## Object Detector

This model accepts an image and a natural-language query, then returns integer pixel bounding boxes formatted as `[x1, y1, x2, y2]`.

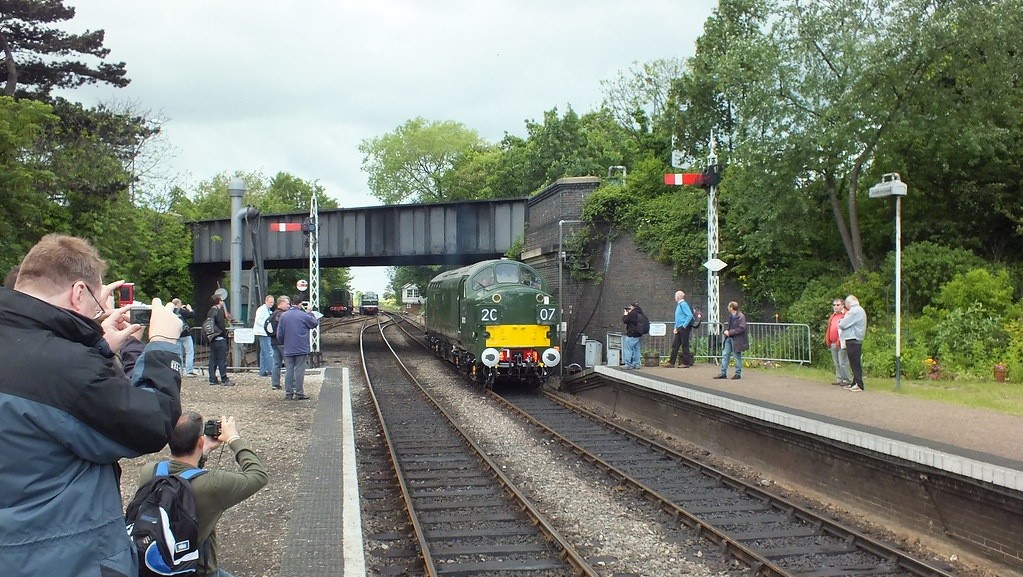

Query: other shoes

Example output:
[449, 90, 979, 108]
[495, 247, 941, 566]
[663, 363, 674, 368]
[223, 381, 238, 385]
[188, 373, 197, 376]
[832, 382, 841, 385]
[296, 395, 309, 400]
[622, 366, 631, 369]
[209, 381, 218, 385]
[844, 385, 851, 389]
[272, 385, 281, 389]
[840, 383, 848, 386]
[632, 366, 639, 369]
[263, 372, 267, 376]
[286, 396, 292, 400]
[678, 364, 689, 368]
[732, 375, 740, 379]
[713, 374, 725, 379]
[850, 384, 861, 391]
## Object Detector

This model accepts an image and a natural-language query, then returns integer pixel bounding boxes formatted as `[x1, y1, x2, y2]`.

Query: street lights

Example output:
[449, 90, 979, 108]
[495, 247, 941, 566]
[869, 173, 907, 391]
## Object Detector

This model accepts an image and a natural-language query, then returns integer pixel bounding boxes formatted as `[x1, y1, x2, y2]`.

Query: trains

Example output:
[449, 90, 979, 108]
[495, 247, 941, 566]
[422, 258, 562, 392]
[359, 291, 379, 316]
[326, 288, 354, 316]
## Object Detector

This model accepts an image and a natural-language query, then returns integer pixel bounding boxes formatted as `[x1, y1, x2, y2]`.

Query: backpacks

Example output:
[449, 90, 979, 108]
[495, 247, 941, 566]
[176, 308, 189, 332]
[126, 461, 209, 577]
[631, 310, 648, 334]
[202, 309, 224, 342]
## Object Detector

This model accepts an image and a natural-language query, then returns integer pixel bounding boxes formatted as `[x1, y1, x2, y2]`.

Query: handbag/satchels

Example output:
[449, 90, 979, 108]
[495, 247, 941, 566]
[680, 301, 703, 328]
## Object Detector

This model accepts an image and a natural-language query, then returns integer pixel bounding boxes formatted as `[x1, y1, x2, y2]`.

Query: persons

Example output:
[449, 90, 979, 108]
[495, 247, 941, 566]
[621, 302, 642, 370]
[277, 296, 318, 401]
[0, 235, 184, 577]
[713, 302, 749, 379]
[253, 295, 274, 376]
[838, 295, 866, 391]
[269, 296, 296, 390]
[207, 294, 235, 386]
[662, 291, 693, 368]
[4, 264, 146, 379]
[137, 411, 269, 577]
[825, 299, 850, 386]
[171, 298, 199, 376]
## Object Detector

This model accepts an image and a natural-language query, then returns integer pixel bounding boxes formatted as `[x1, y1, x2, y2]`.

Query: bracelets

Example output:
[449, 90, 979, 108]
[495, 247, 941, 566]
[227, 436, 240, 445]
[147, 335, 181, 341]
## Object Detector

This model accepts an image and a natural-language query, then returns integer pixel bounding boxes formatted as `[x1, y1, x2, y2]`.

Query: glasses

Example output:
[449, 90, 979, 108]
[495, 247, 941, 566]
[73, 284, 104, 320]
[833, 304, 841, 306]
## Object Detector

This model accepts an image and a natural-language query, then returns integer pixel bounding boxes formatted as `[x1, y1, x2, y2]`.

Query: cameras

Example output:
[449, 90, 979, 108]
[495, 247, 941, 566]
[130, 307, 151, 326]
[203, 420, 223, 435]
[625, 308, 631, 311]
[118, 283, 135, 307]
